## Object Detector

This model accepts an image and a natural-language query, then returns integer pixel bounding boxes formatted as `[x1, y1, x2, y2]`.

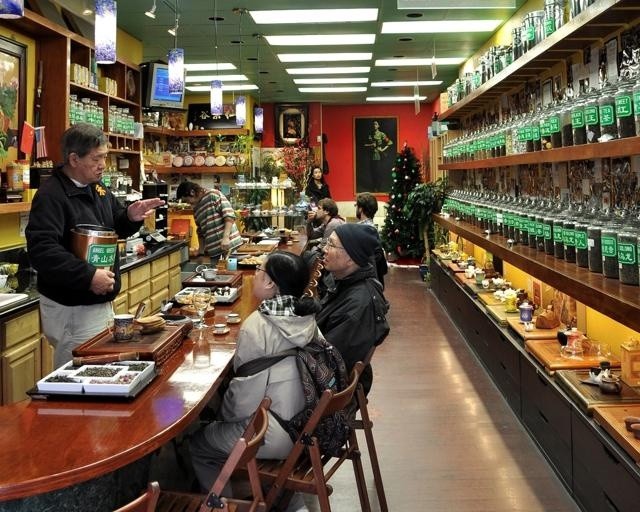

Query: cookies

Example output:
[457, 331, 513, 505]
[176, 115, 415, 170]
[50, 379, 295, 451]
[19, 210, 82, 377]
[137, 317, 166, 335]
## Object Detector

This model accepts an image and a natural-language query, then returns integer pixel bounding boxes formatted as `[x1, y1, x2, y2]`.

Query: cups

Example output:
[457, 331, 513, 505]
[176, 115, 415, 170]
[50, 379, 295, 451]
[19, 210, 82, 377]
[555, 326, 611, 361]
[105, 314, 135, 342]
[229, 314, 239, 322]
[292, 231, 299, 242]
[252, 210, 286, 217]
[215, 323, 225, 333]
[631, 423, 640, 440]
[287, 241, 293, 245]
[623, 415, 640, 431]
[589, 361, 610, 382]
[204, 270, 216, 281]
[227, 258, 237, 271]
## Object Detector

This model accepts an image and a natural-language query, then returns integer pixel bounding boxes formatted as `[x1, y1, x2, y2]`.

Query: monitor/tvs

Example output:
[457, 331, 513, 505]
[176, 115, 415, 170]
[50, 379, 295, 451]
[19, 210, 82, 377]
[137, 60, 185, 110]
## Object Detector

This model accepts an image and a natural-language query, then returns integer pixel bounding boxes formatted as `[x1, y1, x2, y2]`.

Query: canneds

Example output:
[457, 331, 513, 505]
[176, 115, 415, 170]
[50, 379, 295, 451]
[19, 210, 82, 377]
[6, 165, 24, 192]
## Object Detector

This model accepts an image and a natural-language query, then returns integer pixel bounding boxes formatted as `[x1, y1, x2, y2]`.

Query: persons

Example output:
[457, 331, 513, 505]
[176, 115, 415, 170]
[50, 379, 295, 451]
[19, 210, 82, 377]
[354, 192, 379, 228]
[175, 179, 244, 266]
[292, 223, 390, 409]
[286, 116, 300, 137]
[305, 165, 333, 219]
[305, 200, 343, 267]
[25, 124, 167, 373]
[363, 121, 394, 174]
[188, 251, 341, 498]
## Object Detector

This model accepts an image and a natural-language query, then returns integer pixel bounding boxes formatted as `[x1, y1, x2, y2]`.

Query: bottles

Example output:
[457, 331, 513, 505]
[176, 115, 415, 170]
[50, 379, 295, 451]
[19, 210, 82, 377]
[100, 166, 132, 194]
[446, 188, 640, 289]
[14, 160, 30, 189]
[218, 259, 225, 270]
[443, 0, 640, 166]
[71, 94, 135, 137]
[619, 339, 640, 388]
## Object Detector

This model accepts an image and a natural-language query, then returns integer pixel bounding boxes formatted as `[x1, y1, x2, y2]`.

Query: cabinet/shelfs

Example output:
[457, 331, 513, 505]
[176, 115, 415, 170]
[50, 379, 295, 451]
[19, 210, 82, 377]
[143, 125, 252, 174]
[425, 1, 640, 510]
[0, 244, 182, 396]
[41, 37, 141, 185]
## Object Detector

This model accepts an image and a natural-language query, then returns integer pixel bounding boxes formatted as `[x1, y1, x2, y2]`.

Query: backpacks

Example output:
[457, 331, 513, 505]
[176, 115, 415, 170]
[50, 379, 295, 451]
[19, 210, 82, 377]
[231, 312, 358, 457]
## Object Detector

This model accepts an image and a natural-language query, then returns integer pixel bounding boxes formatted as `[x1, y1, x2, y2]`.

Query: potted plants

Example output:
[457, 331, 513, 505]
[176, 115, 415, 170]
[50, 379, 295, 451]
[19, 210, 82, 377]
[380, 140, 427, 269]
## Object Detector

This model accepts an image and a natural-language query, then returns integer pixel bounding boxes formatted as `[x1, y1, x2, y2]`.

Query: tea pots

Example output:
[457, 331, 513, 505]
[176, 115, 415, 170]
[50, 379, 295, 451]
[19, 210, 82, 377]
[599, 373, 622, 395]
[493, 289, 537, 323]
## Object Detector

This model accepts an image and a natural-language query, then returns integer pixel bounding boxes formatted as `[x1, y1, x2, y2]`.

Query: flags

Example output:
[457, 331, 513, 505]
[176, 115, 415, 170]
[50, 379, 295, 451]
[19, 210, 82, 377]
[34, 127, 48, 159]
[20, 121, 35, 155]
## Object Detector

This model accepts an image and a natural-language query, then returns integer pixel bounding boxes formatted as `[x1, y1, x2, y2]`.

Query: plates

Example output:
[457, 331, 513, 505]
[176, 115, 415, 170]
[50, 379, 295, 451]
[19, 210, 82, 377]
[36, 359, 155, 395]
[237, 261, 260, 269]
[578, 373, 621, 386]
[212, 328, 230, 335]
[227, 318, 241, 324]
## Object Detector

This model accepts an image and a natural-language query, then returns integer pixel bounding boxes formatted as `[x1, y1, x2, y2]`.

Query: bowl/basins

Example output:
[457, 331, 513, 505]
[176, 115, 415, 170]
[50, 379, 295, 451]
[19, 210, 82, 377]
[0, 261, 21, 291]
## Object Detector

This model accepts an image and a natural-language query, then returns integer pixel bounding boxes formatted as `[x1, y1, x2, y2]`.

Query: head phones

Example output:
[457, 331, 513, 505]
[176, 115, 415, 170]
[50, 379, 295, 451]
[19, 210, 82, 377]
[189, 186, 195, 196]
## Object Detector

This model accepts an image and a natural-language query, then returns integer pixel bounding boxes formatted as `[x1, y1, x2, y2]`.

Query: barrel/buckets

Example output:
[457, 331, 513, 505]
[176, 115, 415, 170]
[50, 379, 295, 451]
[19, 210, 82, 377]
[69, 222, 119, 273]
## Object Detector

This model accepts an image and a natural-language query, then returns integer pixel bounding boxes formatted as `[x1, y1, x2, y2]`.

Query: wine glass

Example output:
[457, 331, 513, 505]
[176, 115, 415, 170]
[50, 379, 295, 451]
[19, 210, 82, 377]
[192, 287, 212, 328]
[192, 328, 212, 369]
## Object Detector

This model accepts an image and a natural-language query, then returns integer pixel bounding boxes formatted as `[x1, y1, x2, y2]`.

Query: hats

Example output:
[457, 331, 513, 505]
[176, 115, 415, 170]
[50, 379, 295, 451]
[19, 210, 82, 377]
[334, 222, 380, 268]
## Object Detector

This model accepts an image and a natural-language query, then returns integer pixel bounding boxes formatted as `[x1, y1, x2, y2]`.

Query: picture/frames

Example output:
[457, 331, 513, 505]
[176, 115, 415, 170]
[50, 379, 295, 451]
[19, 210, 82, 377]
[274, 102, 310, 148]
[353, 116, 401, 197]
[0, 35, 28, 181]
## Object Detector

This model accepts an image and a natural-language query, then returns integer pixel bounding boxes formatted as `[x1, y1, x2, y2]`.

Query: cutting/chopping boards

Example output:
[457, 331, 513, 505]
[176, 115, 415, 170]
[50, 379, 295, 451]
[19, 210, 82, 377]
[237, 243, 275, 252]
